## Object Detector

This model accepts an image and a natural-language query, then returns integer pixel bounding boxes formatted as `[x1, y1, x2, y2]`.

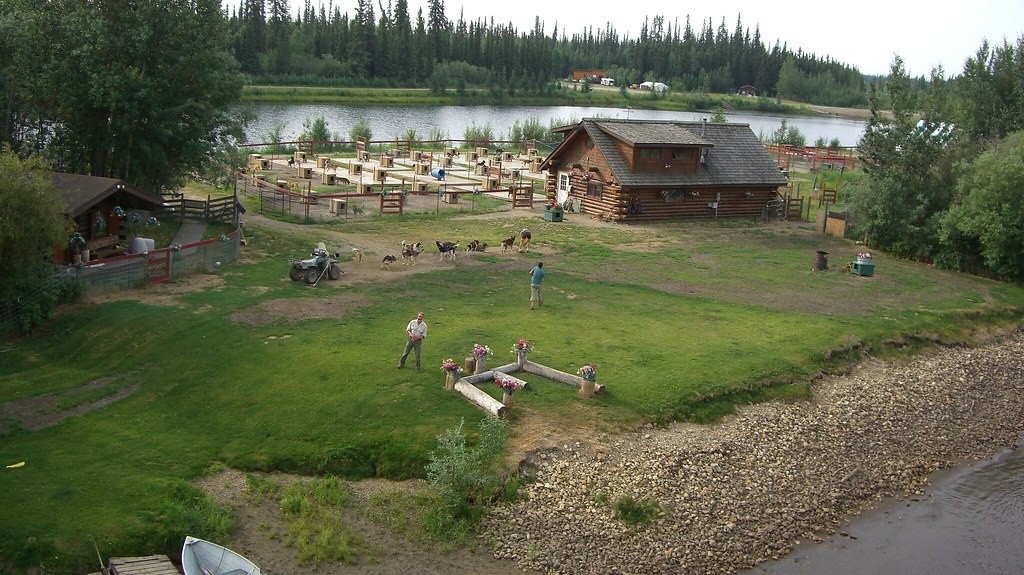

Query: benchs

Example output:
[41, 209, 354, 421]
[85, 234, 124, 258]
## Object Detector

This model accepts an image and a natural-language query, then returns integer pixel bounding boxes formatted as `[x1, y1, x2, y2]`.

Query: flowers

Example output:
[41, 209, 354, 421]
[145, 217, 157, 226]
[473, 343, 494, 359]
[569, 168, 576, 176]
[69, 232, 85, 255]
[110, 206, 126, 217]
[576, 366, 596, 382]
[439, 358, 463, 375]
[604, 178, 614, 183]
[494, 377, 519, 396]
[510, 337, 535, 356]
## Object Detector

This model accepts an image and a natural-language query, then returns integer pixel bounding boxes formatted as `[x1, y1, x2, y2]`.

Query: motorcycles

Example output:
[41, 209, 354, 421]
[289, 251, 341, 287]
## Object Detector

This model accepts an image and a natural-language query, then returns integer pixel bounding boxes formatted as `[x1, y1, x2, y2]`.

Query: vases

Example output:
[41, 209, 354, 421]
[72, 254, 81, 264]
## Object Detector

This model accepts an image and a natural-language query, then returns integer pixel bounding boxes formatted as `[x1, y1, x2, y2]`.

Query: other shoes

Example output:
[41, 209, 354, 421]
[530, 305, 534, 310]
[397, 365, 403, 368]
[538, 301, 543, 305]
[416, 366, 420, 369]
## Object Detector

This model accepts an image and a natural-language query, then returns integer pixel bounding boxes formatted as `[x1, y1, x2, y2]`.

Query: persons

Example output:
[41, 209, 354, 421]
[396, 311, 428, 369]
[529, 262, 546, 310]
[519, 228, 531, 252]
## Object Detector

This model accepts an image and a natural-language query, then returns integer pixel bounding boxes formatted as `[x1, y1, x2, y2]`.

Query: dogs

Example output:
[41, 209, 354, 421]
[254, 164, 261, 172]
[465, 240, 488, 253]
[494, 148, 505, 155]
[401, 239, 424, 267]
[520, 159, 534, 166]
[500, 236, 516, 254]
[325, 160, 338, 174]
[447, 152, 458, 160]
[285, 156, 303, 168]
[379, 254, 396, 270]
[352, 204, 366, 219]
[434, 240, 460, 262]
[286, 179, 300, 190]
[336, 177, 350, 187]
[476, 160, 486, 165]
[359, 153, 370, 164]
